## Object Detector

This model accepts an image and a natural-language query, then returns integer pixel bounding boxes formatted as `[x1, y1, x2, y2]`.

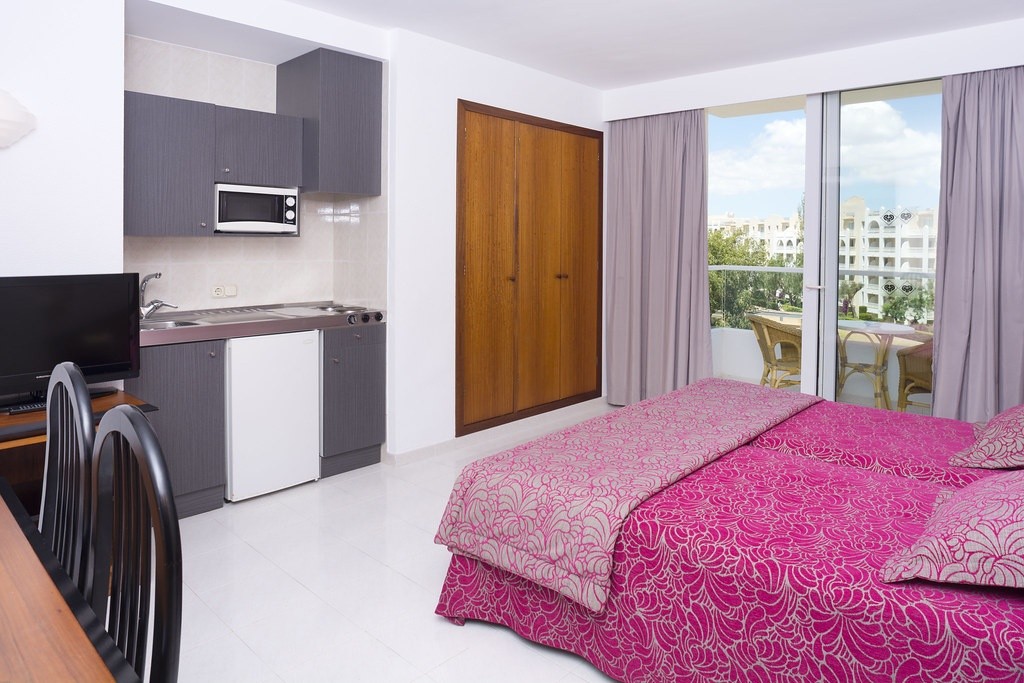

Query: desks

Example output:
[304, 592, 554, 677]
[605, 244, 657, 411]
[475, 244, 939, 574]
[835, 319, 916, 409]
[0, 475, 145, 682]
[0, 387, 162, 450]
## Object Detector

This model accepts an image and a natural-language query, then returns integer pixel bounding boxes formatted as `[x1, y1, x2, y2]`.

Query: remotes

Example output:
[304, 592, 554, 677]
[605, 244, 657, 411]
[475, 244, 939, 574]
[8, 403, 46, 415]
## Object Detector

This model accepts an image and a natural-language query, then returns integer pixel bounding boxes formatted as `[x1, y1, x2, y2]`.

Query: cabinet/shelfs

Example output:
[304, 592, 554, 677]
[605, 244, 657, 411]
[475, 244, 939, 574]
[274, 47, 383, 197]
[121, 339, 225, 524]
[319, 319, 388, 479]
[122, 91, 304, 237]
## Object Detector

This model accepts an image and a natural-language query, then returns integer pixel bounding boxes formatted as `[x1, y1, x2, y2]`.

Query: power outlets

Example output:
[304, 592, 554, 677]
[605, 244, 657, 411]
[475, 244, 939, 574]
[212, 286, 226, 298]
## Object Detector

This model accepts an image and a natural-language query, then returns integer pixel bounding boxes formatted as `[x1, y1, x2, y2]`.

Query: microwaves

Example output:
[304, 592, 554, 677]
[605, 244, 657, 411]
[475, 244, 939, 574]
[214, 183, 299, 234]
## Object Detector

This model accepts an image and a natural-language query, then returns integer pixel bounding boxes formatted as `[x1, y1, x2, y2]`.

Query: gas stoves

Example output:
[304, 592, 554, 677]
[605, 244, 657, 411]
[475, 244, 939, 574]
[307, 303, 387, 330]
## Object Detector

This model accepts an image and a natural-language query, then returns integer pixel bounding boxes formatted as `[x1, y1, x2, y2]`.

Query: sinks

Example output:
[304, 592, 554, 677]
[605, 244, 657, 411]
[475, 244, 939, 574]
[138, 320, 201, 330]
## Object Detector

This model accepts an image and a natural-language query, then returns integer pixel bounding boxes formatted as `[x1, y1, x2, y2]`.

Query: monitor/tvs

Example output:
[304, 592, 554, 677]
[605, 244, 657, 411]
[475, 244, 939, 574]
[0, 272, 140, 412]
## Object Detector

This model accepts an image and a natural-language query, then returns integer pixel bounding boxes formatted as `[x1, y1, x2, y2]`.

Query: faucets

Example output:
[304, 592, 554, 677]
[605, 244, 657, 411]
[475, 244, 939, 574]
[138, 272, 179, 320]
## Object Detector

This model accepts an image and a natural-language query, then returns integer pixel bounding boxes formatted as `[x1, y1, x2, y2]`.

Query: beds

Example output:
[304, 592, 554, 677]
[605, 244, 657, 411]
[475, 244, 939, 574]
[433, 377, 1024, 683]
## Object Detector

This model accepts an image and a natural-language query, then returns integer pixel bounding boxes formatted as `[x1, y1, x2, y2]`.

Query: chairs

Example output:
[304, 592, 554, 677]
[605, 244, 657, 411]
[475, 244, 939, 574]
[745, 312, 934, 413]
[32, 360, 93, 596]
[79, 404, 184, 683]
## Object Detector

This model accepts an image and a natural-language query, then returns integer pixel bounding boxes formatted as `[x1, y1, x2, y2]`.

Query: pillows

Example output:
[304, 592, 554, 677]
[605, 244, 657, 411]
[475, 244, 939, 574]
[948, 402, 1024, 469]
[877, 470, 1024, 591]
